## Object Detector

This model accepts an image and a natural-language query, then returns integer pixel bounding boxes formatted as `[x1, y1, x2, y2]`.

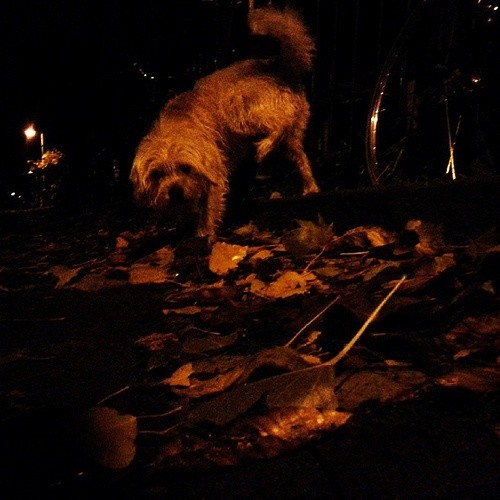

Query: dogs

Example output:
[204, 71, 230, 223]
[127, 4, 321, 249]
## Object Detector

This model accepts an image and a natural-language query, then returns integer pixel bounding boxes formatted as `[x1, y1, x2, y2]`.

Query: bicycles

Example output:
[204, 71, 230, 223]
[360, 0, 499, 189]
